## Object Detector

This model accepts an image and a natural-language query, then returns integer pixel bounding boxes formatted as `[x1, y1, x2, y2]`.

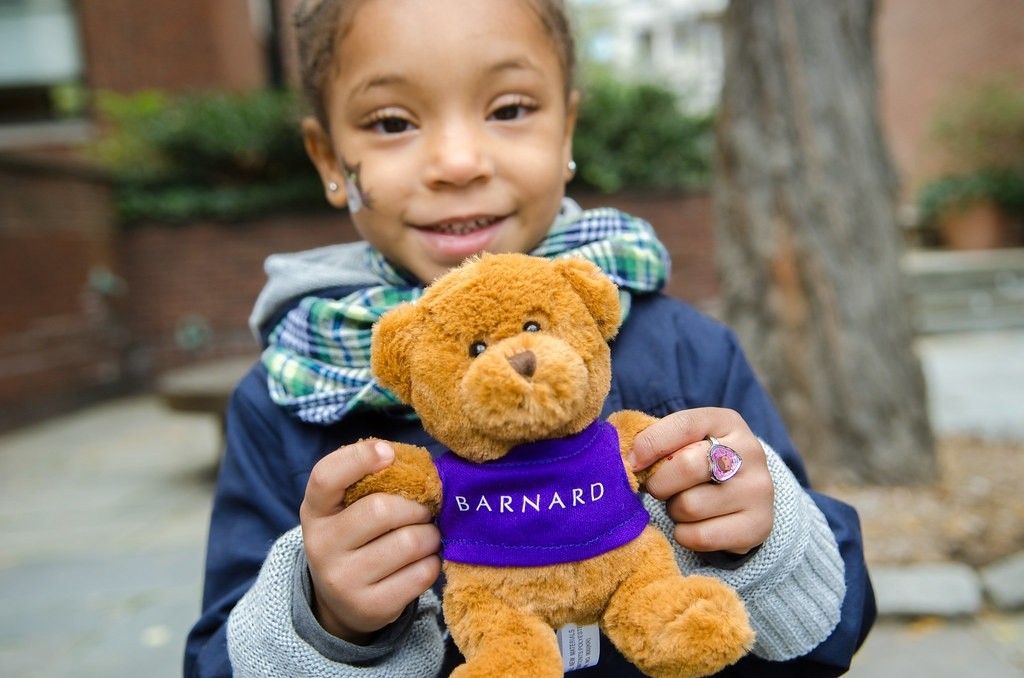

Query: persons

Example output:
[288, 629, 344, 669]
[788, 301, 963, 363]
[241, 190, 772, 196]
[182, 0, 876, 678]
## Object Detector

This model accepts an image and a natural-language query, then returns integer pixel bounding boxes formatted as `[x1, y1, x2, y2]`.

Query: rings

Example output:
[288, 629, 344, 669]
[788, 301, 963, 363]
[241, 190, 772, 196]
[707, 435, 741, 483]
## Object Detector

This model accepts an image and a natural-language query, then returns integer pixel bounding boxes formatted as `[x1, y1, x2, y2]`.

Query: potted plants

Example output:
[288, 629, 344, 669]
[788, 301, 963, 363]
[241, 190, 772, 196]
[916, 169, 1018, 249]
[89, 73, 721, 361]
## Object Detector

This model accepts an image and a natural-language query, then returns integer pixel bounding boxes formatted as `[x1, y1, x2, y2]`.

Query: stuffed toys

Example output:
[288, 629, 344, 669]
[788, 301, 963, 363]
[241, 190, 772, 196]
[343, 253, 756, 678]
